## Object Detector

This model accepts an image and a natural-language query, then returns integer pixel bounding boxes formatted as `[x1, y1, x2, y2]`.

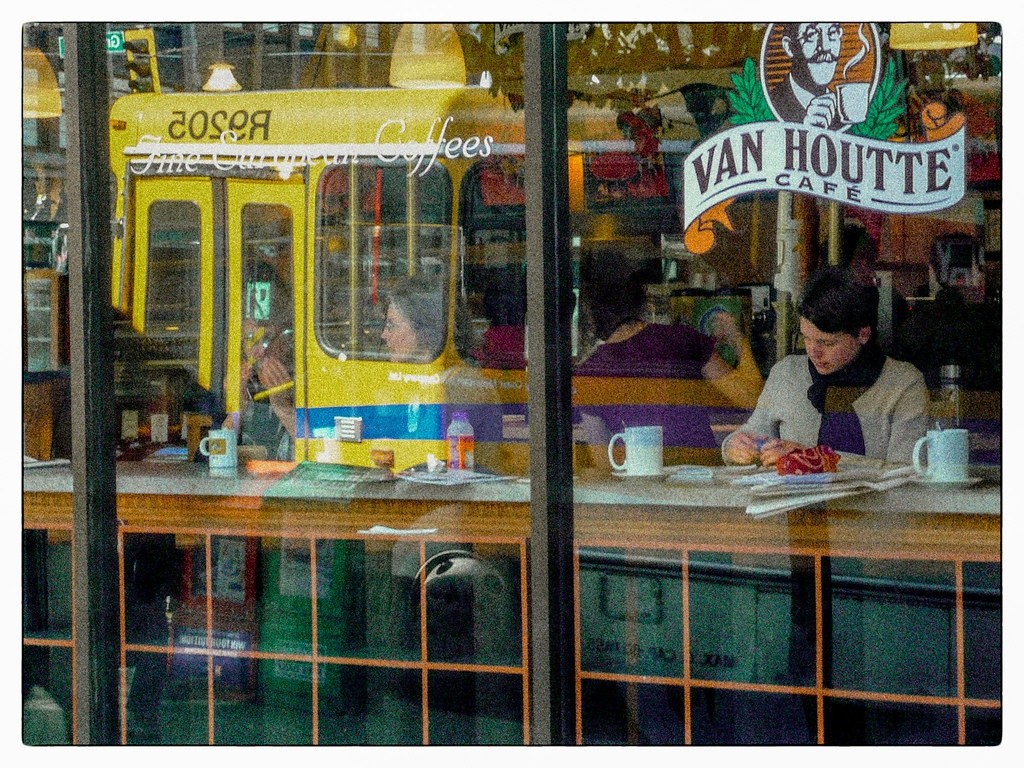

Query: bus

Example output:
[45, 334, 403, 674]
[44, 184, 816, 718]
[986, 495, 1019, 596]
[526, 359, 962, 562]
[96, 85, 1002, 746]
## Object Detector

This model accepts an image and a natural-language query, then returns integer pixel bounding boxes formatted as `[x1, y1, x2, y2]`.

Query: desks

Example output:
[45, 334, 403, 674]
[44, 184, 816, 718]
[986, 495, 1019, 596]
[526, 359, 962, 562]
[23, 457, 1002, 746]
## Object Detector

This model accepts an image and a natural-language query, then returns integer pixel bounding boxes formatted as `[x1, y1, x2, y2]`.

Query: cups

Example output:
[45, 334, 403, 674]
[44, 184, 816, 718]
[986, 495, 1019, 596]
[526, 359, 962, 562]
[199, 431, 238, 468]
[608, 426, 663, 474]
[940, 365, 962, 426]
[912, 430, 968, 478]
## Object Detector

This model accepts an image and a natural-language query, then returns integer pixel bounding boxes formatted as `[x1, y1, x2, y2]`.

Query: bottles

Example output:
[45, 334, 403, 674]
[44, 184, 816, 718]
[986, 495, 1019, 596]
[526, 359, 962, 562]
[446, 412, 474, 473]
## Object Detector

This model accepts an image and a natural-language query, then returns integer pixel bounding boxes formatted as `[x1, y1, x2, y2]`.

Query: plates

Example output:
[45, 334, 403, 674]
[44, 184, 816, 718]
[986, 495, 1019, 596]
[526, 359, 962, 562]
[612, 473, 662, 479]
[910, 478, 982, 489]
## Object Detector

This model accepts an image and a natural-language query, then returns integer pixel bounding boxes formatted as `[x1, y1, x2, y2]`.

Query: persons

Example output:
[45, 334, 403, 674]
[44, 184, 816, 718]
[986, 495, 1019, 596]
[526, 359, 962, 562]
[818, 224, 921, 376]
[48, 307, 297, 736]
[570, 273, 764, 447]
[900, 232, 1002, 394]
[466, 277, 527, 371]
[720, 273, 929, 474]
[381, 276, 469, 365]
